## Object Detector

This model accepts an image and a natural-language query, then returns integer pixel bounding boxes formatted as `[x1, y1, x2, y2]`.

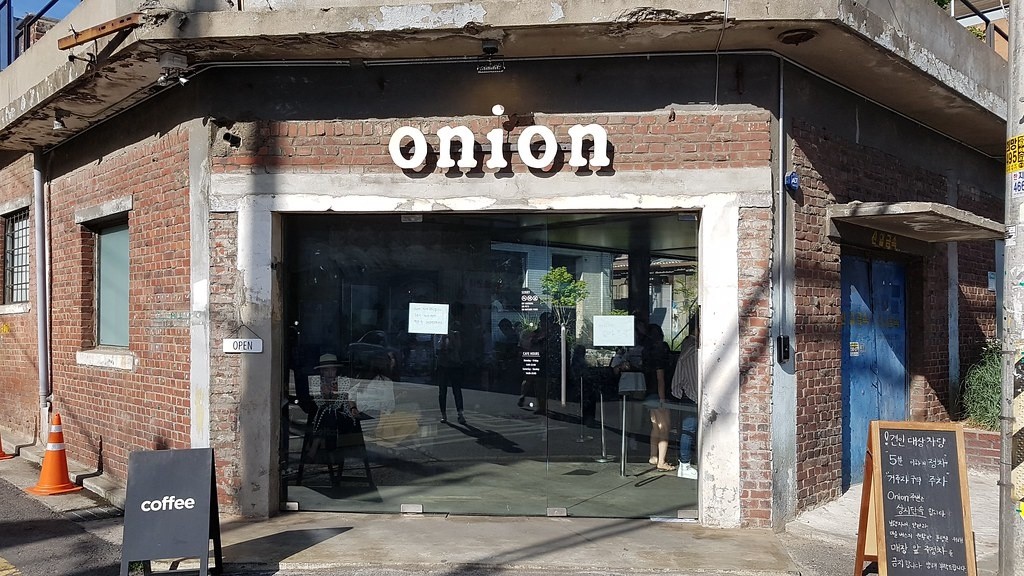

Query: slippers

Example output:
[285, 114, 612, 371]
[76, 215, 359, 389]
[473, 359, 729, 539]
[649, 458, 659, 465]
[659, 464, 676, 473]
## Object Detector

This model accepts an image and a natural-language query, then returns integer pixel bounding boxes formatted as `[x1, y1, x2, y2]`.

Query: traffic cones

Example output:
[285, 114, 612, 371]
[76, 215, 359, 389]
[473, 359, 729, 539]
[23, 413, 85, 496]
[0, 430, 14, 460]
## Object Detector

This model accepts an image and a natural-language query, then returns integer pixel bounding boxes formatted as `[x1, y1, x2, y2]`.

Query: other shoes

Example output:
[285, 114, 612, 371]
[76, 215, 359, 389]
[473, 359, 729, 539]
[458, 415, 467, 424]
[517, 396, 525, 407]
[441, 417, 447, 423]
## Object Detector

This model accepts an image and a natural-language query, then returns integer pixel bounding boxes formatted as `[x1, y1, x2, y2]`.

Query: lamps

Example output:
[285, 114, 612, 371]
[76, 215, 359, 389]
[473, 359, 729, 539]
[52, 114, 67, 131]
[157, 72, 190, 87]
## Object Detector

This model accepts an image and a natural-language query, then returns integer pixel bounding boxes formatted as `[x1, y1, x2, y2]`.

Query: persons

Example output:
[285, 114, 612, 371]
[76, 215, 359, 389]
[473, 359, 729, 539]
[434, 327, 466, 424]
[498, 312, 597, 428]
[610, 322, 641, 451]
[671, 315, 698, 481]
[348, 349, 395, 423]
[296, 354, 360, 465]
[641, 324, 676, 472]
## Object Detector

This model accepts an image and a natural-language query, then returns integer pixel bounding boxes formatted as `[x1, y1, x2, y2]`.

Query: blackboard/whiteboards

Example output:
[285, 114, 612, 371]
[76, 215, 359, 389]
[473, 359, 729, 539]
[871, 419, 979, 576]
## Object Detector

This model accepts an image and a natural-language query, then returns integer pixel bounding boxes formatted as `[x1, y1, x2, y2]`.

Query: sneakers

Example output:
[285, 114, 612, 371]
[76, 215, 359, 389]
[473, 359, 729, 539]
[676, 460, 698, 479]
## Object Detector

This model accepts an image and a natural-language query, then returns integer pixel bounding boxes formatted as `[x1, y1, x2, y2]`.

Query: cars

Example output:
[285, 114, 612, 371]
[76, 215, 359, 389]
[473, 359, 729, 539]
[346, 329, 407, 377]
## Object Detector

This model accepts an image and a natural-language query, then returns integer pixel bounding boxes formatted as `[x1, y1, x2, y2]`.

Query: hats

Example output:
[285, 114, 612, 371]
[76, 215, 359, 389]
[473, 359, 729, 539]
[314, 354, 344, 371]
